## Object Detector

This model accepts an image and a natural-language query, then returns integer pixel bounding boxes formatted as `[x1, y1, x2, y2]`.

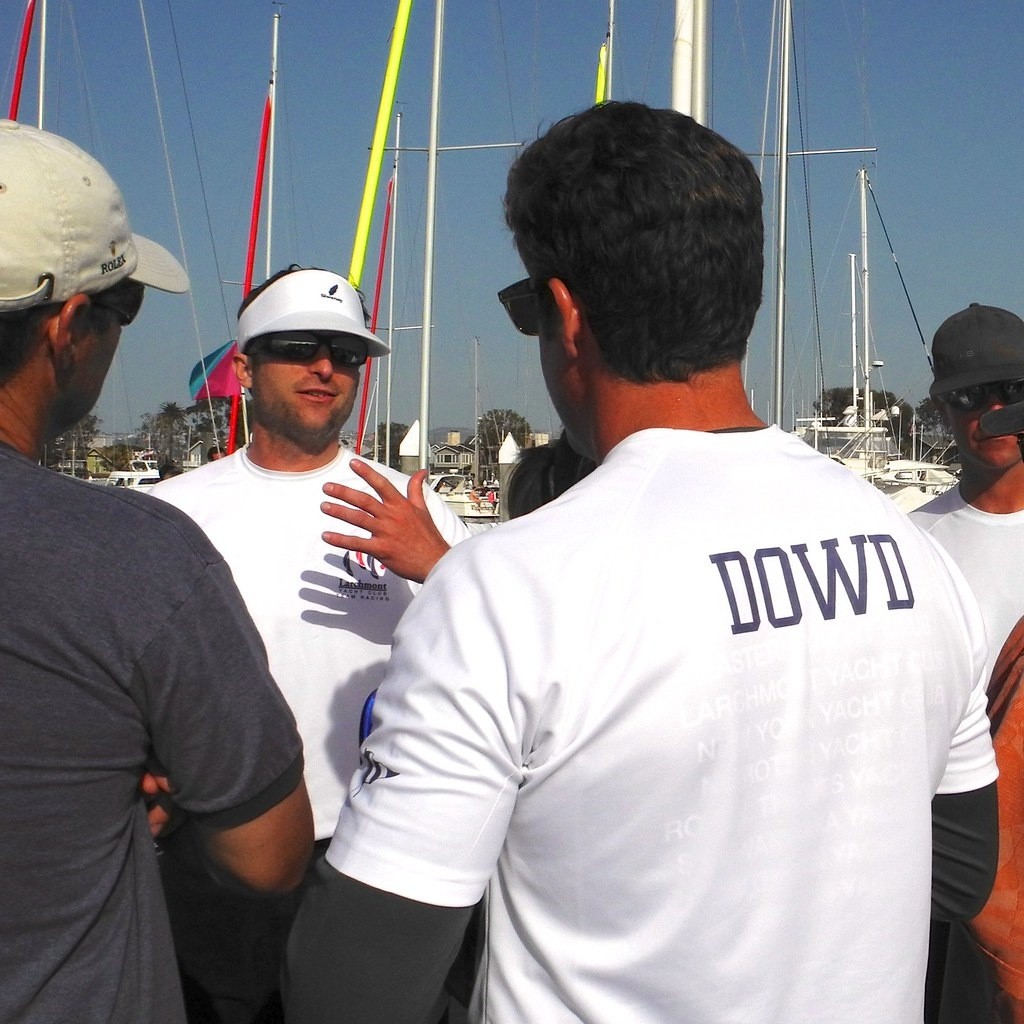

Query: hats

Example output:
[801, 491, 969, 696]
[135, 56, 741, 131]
[0, 119, 190, 311]
[980, 401, 1024, 436]
[237, 270, 391, 361]
[930, 303, 1024, 394]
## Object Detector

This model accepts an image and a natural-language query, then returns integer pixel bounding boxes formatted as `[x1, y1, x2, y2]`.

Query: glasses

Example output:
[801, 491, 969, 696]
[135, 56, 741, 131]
[936, 379, 1024, 411]
[246, 334, 368, 367]
[92, 282, 145, 326]
[498, 278, 548, 336]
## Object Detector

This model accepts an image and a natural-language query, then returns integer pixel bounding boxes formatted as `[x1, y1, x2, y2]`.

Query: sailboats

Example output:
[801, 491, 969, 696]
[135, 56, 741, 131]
[6, 0, 963, 544]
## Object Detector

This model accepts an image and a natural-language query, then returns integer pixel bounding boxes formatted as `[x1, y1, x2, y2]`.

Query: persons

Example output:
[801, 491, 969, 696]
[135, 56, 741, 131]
[0, 120, 1023, 1024]
[276, 102, 1000, 1023]
